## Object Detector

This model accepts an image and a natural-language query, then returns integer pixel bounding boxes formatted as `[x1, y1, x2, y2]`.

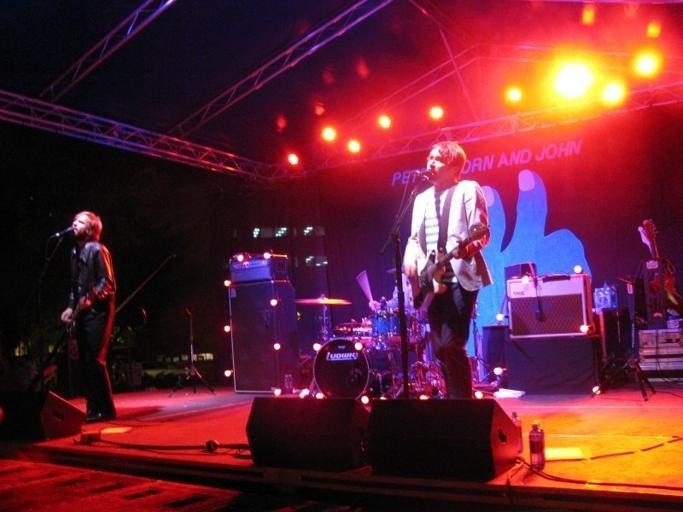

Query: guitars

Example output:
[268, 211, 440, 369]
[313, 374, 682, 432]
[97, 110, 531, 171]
[643, 219, 683, 316]
[64, 277, 114, 338]
[407, 223, 487, 312]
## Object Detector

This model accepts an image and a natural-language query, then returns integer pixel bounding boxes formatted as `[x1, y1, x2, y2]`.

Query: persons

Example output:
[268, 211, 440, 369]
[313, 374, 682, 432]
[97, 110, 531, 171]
[58, 207, 118, 421]
[366, 276, 414, 315]
[401, 139, 492, 401]
[59, 332, 80, 360]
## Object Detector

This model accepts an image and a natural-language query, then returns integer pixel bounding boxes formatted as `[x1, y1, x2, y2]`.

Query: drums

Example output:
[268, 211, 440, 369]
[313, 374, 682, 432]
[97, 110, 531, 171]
[334, 321, 374, 339]
[312, 336, 398, 400]
[370, 306, 426, 348]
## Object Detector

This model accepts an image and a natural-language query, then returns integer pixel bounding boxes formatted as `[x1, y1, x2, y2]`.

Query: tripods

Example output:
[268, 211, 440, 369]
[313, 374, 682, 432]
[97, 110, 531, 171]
[592, 259, 657, 401]
[168, 307, 217, 397]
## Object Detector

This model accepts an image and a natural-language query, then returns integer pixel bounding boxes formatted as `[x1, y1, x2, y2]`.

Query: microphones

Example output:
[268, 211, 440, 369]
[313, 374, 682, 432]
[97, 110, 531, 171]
[414, 169, 432, 177]
[535, 301, 542, 321]
[49, 226, 73, 243]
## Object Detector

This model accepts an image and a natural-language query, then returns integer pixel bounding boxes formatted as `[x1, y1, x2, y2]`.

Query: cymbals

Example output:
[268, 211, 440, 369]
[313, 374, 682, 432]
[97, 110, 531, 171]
[296, 298, 351, 308]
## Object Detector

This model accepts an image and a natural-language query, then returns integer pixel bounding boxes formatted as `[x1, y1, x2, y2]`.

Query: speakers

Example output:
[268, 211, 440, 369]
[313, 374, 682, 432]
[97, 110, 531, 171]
[481, 324, 509, 386]
[505, 336, 599, 394]
[613, 276, 648, 355]
[368, 398, 521, 482]
[229, 278, 297, 393]
[246, 397, 371, 470]
[0, 390, 88, 439]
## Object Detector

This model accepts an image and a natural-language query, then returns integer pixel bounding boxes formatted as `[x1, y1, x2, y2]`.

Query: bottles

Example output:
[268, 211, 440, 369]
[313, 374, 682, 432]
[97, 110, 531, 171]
[510, 411, 523, 455]
[528, 422, 545, 469]
[593, 286, 618, 309]
